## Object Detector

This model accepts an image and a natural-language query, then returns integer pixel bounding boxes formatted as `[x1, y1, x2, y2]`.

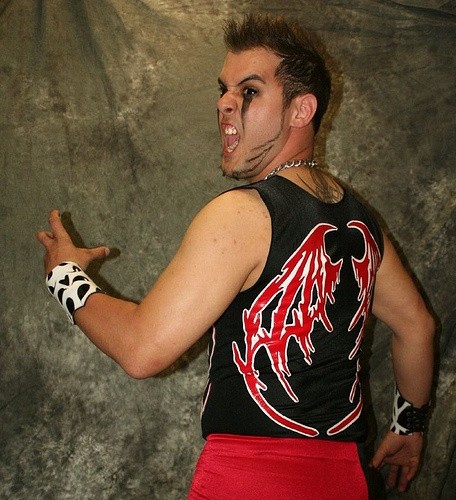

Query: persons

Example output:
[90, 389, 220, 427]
[35, 8, 440, 500]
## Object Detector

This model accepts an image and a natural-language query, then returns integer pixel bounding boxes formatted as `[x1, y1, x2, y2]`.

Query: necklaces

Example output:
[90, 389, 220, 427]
[262, 159, 320, 181]
